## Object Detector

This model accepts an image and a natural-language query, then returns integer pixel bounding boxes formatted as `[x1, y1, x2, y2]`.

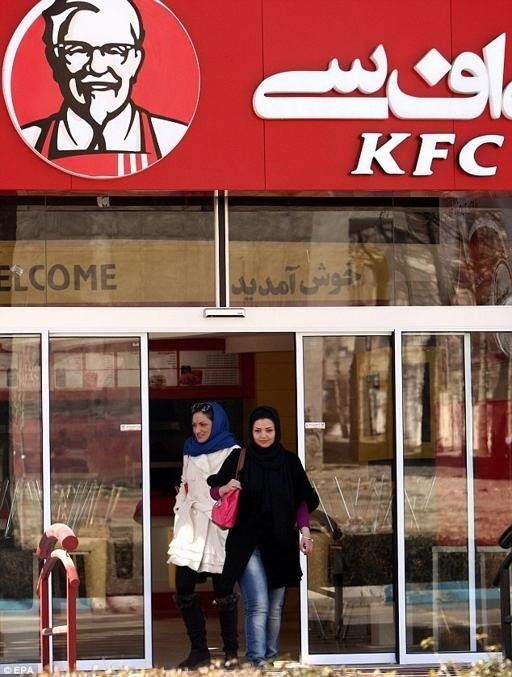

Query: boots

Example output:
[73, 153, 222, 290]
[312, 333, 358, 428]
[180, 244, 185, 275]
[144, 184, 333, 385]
[217, 605, 241, 670]
[177, 603, 211, 670]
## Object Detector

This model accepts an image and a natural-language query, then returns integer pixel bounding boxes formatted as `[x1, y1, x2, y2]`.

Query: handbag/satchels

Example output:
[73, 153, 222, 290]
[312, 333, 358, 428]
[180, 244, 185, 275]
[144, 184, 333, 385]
[211, 485, 243, 531]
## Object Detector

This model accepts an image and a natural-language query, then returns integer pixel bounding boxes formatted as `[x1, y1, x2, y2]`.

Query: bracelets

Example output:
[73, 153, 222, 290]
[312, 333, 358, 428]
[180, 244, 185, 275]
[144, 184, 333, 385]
[301, 534, 314, 543]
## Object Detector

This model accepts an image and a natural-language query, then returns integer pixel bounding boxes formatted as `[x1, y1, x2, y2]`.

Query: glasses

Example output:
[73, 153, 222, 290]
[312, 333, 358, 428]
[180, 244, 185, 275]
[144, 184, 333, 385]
[191, 402, 211, 413]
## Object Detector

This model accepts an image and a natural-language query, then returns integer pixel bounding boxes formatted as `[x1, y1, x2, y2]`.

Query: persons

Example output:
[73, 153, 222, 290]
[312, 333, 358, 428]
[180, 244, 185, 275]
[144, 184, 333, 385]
[165, 399, 242, 671]
[204, 403, 320, 673]
[13, 1, 188, 162]
